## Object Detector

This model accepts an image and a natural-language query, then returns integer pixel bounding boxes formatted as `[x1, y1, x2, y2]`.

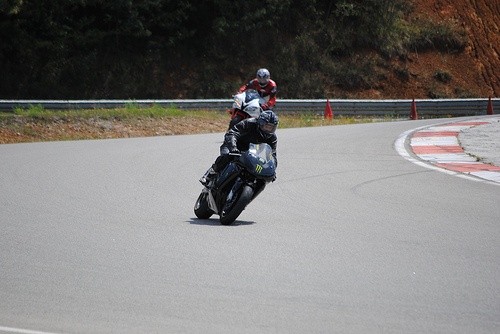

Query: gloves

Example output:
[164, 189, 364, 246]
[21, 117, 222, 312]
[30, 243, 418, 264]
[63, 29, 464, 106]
[230, 147, 240, 153]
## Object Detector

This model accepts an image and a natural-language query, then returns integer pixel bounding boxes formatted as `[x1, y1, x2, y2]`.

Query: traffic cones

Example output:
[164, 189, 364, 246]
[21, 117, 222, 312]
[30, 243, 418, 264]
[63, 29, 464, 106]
[323, 98, 334, 121]
[486, 95, 494, 116]
[410, 99, 418, 120]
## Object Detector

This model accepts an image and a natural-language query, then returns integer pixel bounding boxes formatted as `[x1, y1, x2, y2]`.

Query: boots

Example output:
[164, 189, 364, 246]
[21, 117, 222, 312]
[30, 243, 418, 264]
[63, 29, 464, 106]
[199, 167, 216, 189]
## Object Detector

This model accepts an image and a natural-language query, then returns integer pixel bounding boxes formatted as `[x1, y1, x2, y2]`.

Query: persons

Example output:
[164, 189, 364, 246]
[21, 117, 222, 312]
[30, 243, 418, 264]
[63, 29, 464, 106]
[226, 68, 277, 110]
[199, 110, 279, 187]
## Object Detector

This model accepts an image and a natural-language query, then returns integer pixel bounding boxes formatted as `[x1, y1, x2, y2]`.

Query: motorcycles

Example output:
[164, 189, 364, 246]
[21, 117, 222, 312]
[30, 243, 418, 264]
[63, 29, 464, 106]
[227, 87, 267, 129]
[193, 141, 277, 226]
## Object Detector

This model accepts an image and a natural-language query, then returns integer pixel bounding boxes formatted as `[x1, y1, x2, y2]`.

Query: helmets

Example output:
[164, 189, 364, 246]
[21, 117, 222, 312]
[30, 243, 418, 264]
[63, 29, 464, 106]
[258, 110, 279, 139]
[257, 68, 270, 88]
